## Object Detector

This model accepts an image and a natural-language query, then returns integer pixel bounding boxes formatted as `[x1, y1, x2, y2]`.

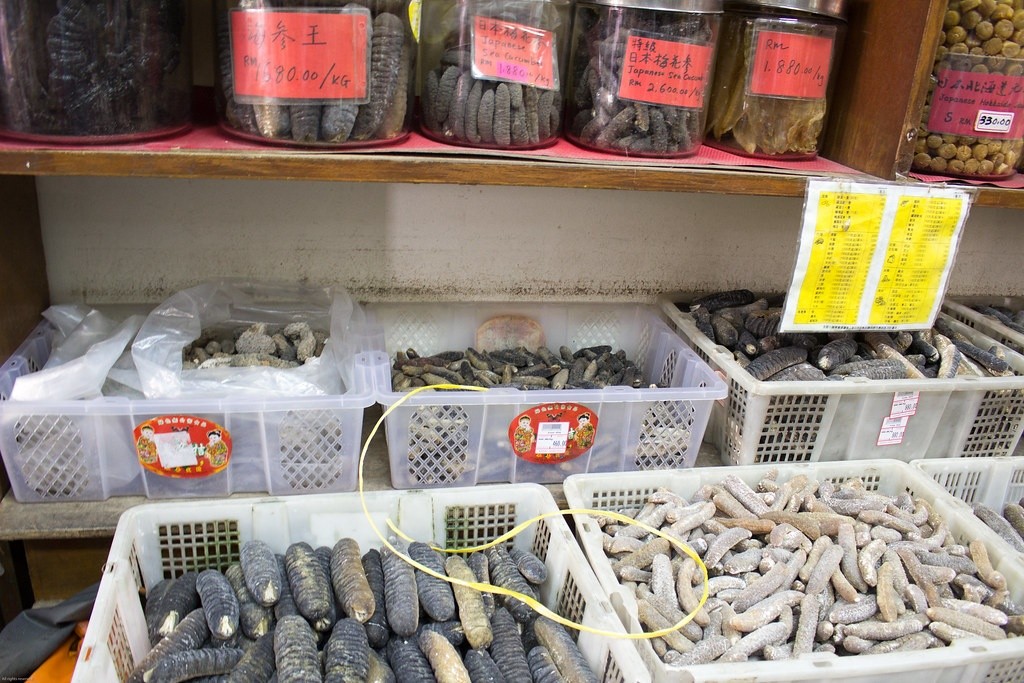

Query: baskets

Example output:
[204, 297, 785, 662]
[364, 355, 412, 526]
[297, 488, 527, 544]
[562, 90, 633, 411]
[0, 318, 376, 504]
[659, 297, 1024, 465]
[368, 301, 728, 489]
[71, 456, 1024, 683]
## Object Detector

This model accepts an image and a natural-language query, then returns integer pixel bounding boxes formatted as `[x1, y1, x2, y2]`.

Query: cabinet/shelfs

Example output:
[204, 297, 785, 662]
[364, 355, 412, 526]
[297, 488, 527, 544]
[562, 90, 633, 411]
[0, 2, 1024, 208]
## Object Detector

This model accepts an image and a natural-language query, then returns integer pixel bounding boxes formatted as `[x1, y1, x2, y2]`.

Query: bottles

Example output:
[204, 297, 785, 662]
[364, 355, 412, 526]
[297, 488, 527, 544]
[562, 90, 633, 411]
[209, 0, 419, 152]
[0, 0, 193, 145]
[703, 0, 849, 160]
[912, 0, 1024, 181]
[419, 0, 576, 149]
[563, 3, 725, 159]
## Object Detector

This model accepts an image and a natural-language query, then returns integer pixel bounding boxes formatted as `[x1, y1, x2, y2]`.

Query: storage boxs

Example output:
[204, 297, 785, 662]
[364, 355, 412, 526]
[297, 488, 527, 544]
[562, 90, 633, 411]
[0, 303, 376, 502]
[909, 456, 1024, 561]
[69, 482, 651, 683]
[376, 301, 728, 491]
[562, 459, 1024, 683]
[658, 293, 1024, 465]
